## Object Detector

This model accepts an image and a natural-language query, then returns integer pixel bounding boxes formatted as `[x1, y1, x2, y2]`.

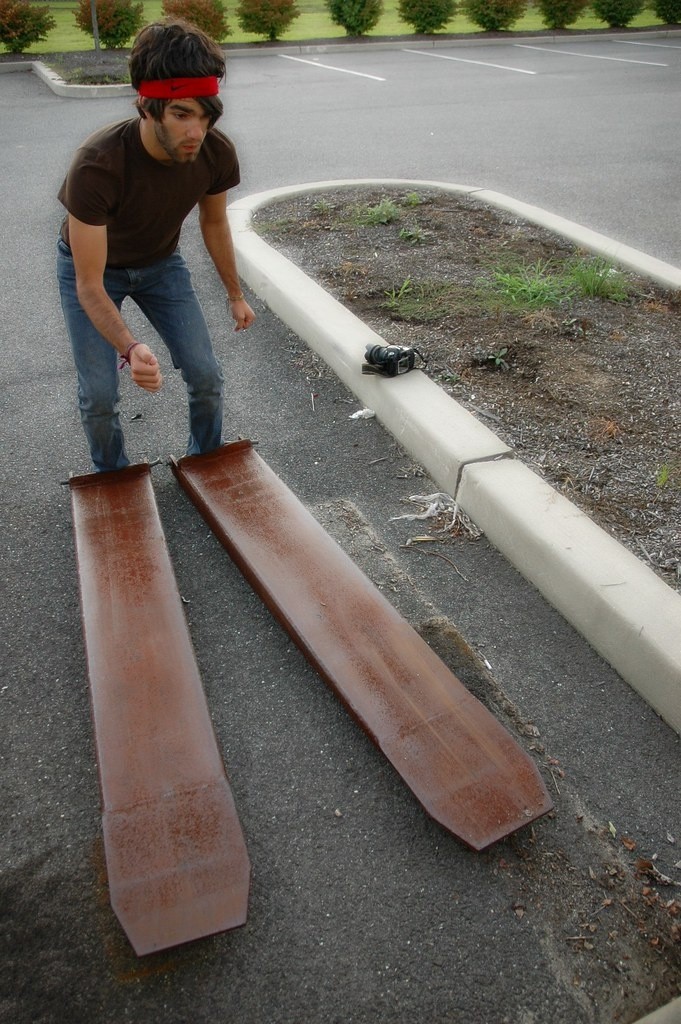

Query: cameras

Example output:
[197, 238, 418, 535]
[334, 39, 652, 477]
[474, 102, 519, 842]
[364, 343, 416, 377]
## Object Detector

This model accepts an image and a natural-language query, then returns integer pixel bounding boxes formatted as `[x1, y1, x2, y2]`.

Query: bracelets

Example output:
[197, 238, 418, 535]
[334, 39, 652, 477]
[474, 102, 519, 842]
[225, 293, 244, 313]
[118, 341, 140, 370]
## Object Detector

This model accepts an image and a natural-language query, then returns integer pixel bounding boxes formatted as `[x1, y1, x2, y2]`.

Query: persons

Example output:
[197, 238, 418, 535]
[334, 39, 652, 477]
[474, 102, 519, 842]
[54, 21, 256, 473]
